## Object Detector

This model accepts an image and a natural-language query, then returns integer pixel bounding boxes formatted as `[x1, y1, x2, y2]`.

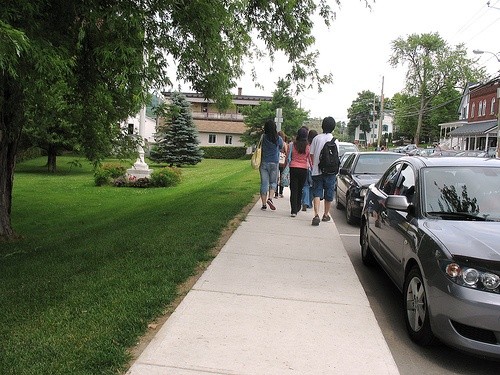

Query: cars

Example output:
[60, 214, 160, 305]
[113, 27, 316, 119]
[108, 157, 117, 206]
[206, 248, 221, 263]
[335, 141, 360, 173]
[359, 156, 500, 364]
[394, 143, 497, 158]
[335, 152, 411, 227]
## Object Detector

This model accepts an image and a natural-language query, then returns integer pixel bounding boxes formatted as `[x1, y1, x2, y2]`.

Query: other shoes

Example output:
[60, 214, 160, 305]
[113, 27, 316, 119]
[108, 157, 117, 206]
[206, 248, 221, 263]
[274, 195, 278, 199]
[291, 212, 297, 217]
[311, 214, 320, 226]
[267, 199, 276, 210]
[261, 205, 267, 210]
[301, 205, 306, 211]
[322, 214, 330, 221]
[279, 193, 284, 198]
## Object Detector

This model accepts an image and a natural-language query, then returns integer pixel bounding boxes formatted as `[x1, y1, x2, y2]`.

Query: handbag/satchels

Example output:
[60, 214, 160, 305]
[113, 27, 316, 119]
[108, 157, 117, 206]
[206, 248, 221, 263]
[251, 134, 263, 170]
[281, 166, 291, 187]
[279, 152, 287, 164]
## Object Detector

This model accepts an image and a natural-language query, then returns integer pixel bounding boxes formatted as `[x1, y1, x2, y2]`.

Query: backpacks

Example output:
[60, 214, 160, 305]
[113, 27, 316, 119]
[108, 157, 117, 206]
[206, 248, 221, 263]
[319, 137, 341, 174]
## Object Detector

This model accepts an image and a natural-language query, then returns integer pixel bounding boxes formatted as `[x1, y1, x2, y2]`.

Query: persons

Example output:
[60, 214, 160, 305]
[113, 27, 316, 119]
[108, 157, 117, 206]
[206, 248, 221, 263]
[309, 116, 340, 226]
[258, 120, 318, 210]
